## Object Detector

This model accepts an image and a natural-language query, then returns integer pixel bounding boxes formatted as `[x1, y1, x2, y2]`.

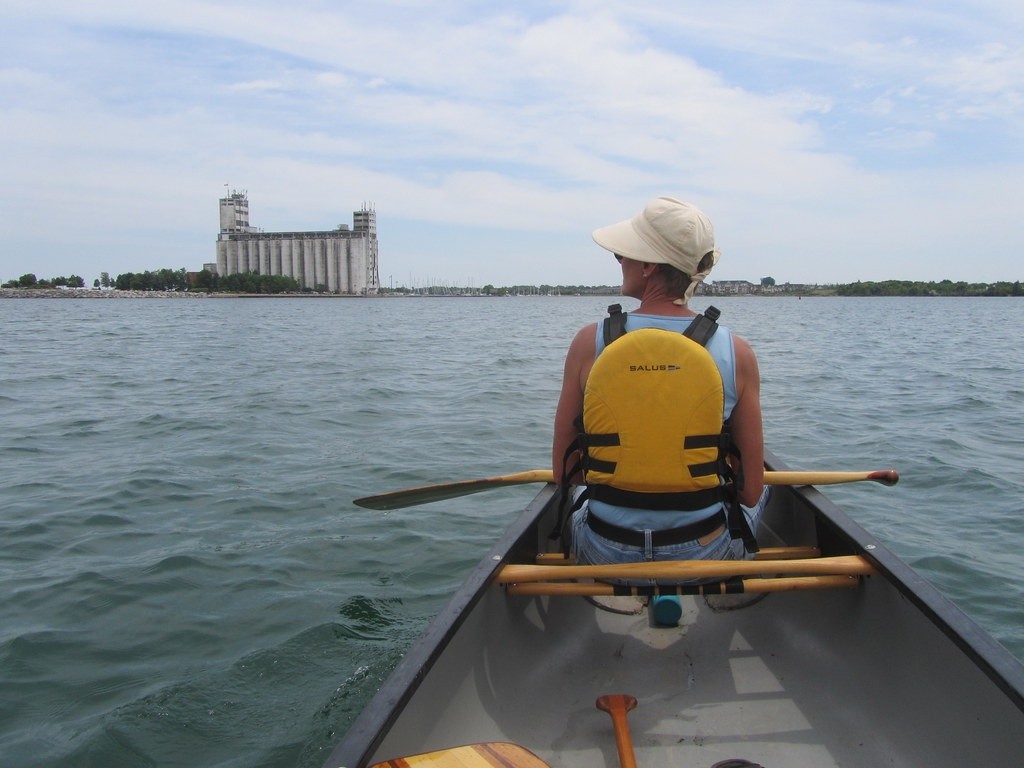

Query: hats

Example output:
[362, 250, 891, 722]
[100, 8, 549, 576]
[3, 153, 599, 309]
[592, 196, 722, 305]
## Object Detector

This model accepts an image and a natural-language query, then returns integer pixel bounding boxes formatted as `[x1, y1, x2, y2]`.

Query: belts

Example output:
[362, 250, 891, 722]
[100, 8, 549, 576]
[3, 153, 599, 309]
[586, 506, 727, 548]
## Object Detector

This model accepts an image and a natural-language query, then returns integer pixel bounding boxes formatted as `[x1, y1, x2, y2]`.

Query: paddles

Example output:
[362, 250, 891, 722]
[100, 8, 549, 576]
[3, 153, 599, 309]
[352, 469, 901, 512]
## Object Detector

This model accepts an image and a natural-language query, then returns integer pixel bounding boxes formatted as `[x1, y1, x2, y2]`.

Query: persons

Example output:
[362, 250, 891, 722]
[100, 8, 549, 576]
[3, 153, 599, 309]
[552, 191, 772, 590]
